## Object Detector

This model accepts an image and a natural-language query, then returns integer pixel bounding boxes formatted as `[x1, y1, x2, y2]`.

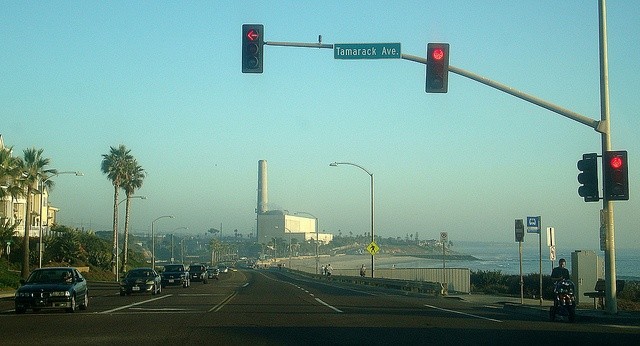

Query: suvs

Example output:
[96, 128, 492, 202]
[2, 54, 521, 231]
[187, 265, 208, 284]
[161, 265, 190, 288]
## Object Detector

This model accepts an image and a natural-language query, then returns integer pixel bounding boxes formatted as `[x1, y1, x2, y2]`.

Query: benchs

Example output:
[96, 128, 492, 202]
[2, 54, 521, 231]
[584, 278, 625, 311]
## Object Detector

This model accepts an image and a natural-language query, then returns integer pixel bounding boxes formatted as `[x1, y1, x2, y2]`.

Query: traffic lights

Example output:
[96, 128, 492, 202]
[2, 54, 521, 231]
[242, 23, 264, 73]
[577, 153, 598, 202]
[603, 150, 628, 201]
[426, 42, 449, 93]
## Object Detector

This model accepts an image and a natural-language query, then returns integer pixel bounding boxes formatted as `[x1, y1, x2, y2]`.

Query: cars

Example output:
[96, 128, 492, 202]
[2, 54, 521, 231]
[206, 268, 220, 280]
[120, 268, 161, 297]
[217, 263, 228, 274]
[14, 268, 89, 313]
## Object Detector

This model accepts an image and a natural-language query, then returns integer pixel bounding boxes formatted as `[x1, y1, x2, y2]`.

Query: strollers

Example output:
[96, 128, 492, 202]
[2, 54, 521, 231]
[548, 279, 575, 323]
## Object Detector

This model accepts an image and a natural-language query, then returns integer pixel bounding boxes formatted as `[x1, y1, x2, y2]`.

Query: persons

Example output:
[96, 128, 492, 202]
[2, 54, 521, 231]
[327, 263, 333, 275]
[359, 263, 367, 277]
[324, 264, 329, 275]
[321, 264, 325, 275]
[551, 259, 570, 306]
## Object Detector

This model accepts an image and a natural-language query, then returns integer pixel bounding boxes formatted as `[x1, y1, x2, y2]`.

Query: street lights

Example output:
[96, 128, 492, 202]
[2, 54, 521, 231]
[151, 215, 176, 268]
[329, 161, 375, 280]
[39, 171, 85, 269]
[275, 226, 291, 271]
[115, 196, 146, 281]
[170, 227, 188, 263]
[294, 211, 319, 276]
